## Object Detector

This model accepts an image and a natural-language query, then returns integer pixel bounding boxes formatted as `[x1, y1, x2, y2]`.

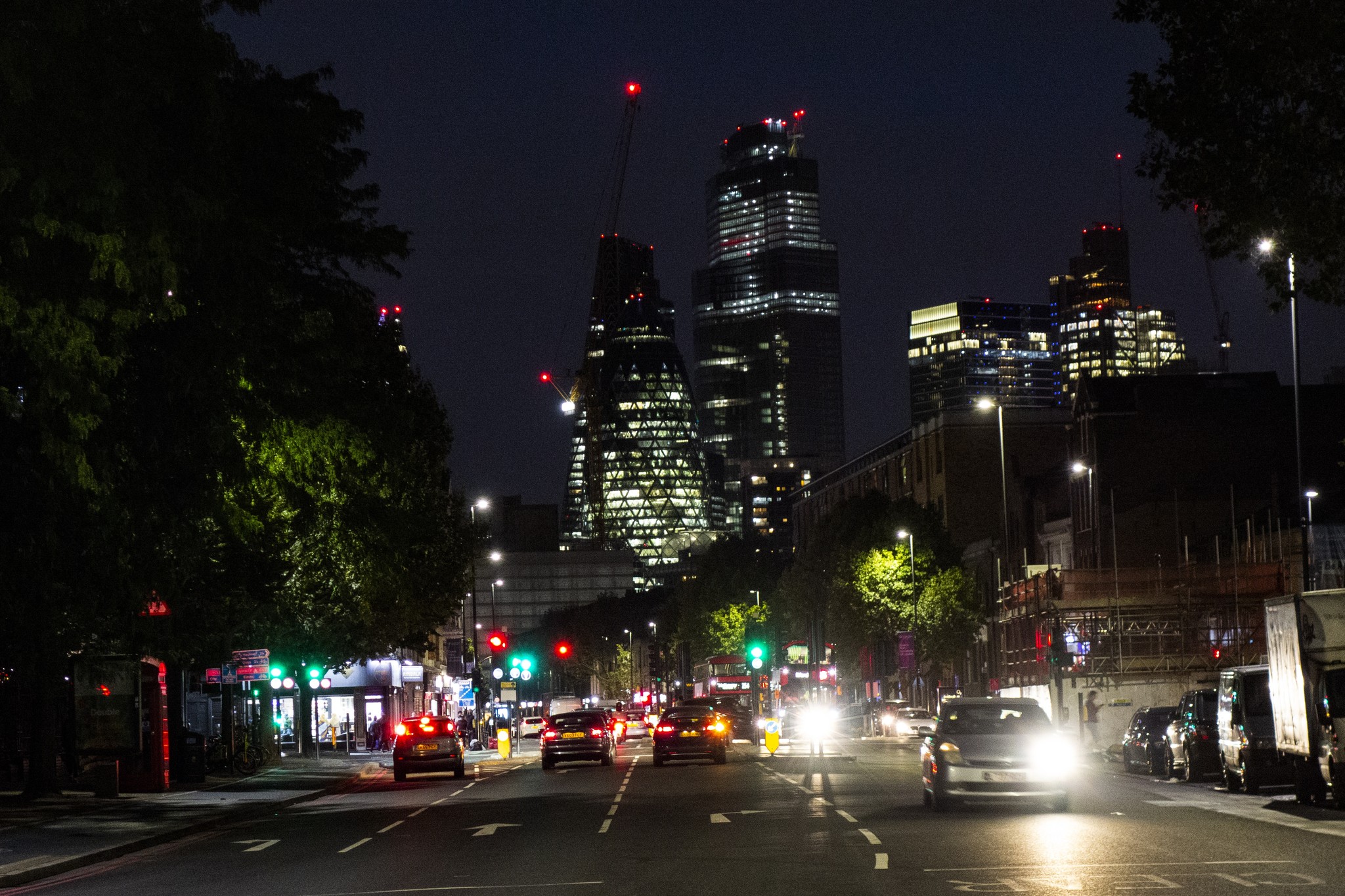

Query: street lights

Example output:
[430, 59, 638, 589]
[1069, 462, 1096, 525]
[976, 397, 1019, 684]
[1302, 490, 1319, 591]
[623, 628, 634, 703]
[649, 621, 661, 715]
[490, 579, 504, 700]
[897, 529, 924, 698]
[1259, 236, 1310, 593]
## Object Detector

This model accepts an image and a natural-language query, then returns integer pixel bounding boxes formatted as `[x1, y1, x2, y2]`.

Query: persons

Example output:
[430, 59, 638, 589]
[367, 715, 389, 752]
[486, 715, 507, 739]
[1085, 691, 1104, 735]
[457, 707, 477, 749]
[674, 696, 685, 722]
[610, 702, 628, 722]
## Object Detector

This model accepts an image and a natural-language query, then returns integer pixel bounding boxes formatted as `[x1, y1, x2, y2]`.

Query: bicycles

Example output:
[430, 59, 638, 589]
[201, 721, 270, 776]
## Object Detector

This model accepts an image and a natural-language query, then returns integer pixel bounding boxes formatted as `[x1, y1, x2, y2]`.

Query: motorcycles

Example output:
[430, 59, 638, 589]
[612, 721, 627, 746]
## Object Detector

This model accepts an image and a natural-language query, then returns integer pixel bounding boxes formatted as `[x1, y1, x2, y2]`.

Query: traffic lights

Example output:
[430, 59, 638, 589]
[648, 652, 661, 678]
[748, 627, 767, 670]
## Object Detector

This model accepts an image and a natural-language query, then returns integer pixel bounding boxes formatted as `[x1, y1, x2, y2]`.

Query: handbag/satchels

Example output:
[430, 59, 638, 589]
[469, 739, 486, 751]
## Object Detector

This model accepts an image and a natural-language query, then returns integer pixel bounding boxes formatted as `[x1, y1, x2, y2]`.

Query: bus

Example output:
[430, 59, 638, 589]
[772, 641, 839, 708]
[692, 654, 752, 709]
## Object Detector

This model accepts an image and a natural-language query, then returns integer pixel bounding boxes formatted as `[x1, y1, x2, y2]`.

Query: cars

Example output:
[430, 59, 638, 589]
[918, 697, 1072, 812]
[625, 709, 649, 738]
[651, 696, 754, 765]
[883, 700, 938, 734]
[540, 707, 617, 769]
[520, 716, 546, 738]
[1123, 687, 1220, 783]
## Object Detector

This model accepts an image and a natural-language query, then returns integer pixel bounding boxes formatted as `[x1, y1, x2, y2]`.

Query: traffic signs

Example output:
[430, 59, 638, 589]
[231, 649, 270, 660]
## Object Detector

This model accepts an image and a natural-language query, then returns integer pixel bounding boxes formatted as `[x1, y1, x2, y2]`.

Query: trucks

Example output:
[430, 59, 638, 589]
[1263, 588, 1345, 811]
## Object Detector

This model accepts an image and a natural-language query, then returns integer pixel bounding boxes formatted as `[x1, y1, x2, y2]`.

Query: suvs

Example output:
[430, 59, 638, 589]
[391, 715, 465, 781]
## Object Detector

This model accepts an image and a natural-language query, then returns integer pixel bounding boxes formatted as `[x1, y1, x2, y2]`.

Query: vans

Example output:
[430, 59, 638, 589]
[1218, 664, 1300, 797]
[596, 699, 620, 711]
[549, 697, 584, 716]
